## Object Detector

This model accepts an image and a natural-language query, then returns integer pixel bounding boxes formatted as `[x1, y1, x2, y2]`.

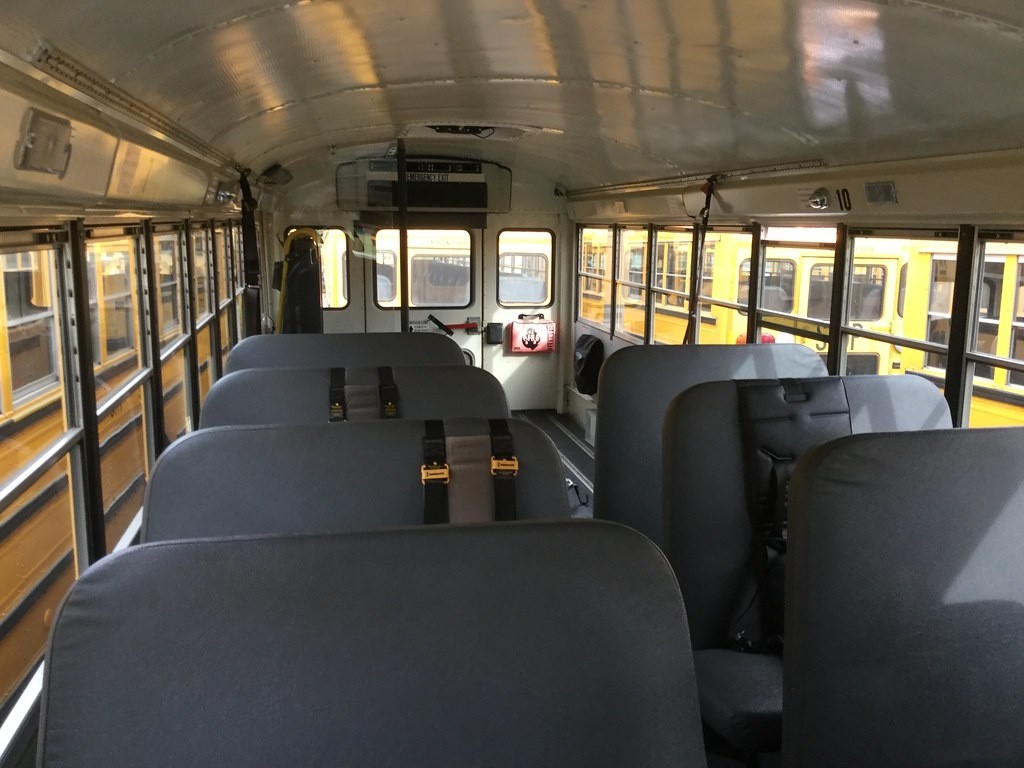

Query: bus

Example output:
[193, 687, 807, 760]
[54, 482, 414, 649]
[578, 227, 1024, 427]
[1, 221, 241, 701]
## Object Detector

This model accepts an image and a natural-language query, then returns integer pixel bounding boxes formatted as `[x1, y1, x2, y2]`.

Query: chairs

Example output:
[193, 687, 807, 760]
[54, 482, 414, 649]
[37, 330, 1024, 768]
